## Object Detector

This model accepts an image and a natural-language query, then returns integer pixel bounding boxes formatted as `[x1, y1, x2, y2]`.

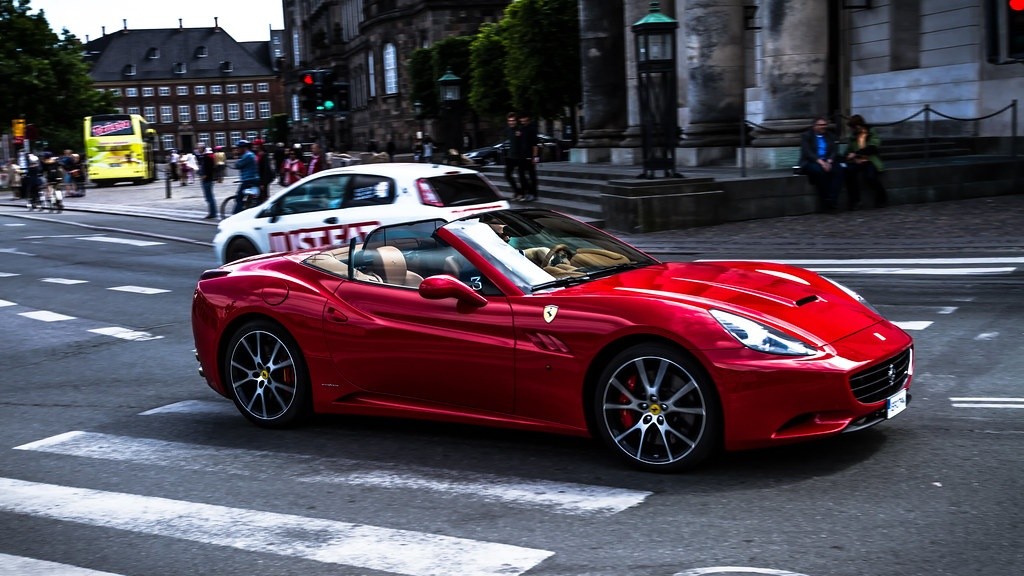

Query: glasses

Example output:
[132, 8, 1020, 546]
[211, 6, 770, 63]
[494, 232, 506, 239]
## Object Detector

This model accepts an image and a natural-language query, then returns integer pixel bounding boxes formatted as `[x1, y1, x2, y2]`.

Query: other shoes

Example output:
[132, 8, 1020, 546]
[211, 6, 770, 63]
[205, 214, 217, 219]
[512, 194, 537, 202]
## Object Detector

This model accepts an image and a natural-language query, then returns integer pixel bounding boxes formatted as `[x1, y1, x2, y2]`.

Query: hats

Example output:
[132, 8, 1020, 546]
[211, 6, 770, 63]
[292, 143, 302, 149]
[202, 147, 213, 154]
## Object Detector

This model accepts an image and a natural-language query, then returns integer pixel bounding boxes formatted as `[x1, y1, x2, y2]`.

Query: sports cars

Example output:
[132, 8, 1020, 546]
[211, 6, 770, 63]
[191, 206, 915, 473]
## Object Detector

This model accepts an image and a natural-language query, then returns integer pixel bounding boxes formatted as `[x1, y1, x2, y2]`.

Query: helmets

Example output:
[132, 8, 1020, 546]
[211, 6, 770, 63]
[213, 145, 222, 151]
[252, 139, 264, 145]
[234, 140, 251, 147]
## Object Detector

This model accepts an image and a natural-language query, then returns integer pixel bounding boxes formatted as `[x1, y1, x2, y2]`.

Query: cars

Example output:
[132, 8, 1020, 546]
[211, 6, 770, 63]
[213, 163, 511, 267]
[463, 133, 572, 163]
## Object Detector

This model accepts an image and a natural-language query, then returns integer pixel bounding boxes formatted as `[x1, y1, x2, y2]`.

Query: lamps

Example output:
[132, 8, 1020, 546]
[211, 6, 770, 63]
[743, 4, 762, 30]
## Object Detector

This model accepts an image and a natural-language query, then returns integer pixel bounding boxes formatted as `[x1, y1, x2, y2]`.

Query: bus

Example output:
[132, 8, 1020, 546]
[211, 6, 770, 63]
[82, 112, 158, 186]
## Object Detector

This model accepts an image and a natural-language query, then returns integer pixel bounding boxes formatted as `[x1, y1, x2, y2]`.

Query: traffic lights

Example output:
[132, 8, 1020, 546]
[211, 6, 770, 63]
[293, 69, 351, 120]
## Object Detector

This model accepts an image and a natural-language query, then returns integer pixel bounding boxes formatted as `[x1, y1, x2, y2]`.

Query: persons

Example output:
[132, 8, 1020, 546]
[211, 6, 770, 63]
[797, 116, 849, 213]
[213, 146, 228, 183]
[7, 148, 88, 204]
[275, 141, 327, 186]
[168, 148, 197, 186]
[230, 138, 278, 212]
[502, 113, 540, 202]
[841, 115, 887, 208]
[415, 137, 438, 164]
[193, 142, 219, 219]
[461, 223, 553, 291]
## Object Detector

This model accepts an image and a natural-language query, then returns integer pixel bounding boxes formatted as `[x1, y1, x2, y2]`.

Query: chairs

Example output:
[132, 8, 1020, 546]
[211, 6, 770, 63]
[373, 246, 422, 285]
[443, 255, 463, 280]
